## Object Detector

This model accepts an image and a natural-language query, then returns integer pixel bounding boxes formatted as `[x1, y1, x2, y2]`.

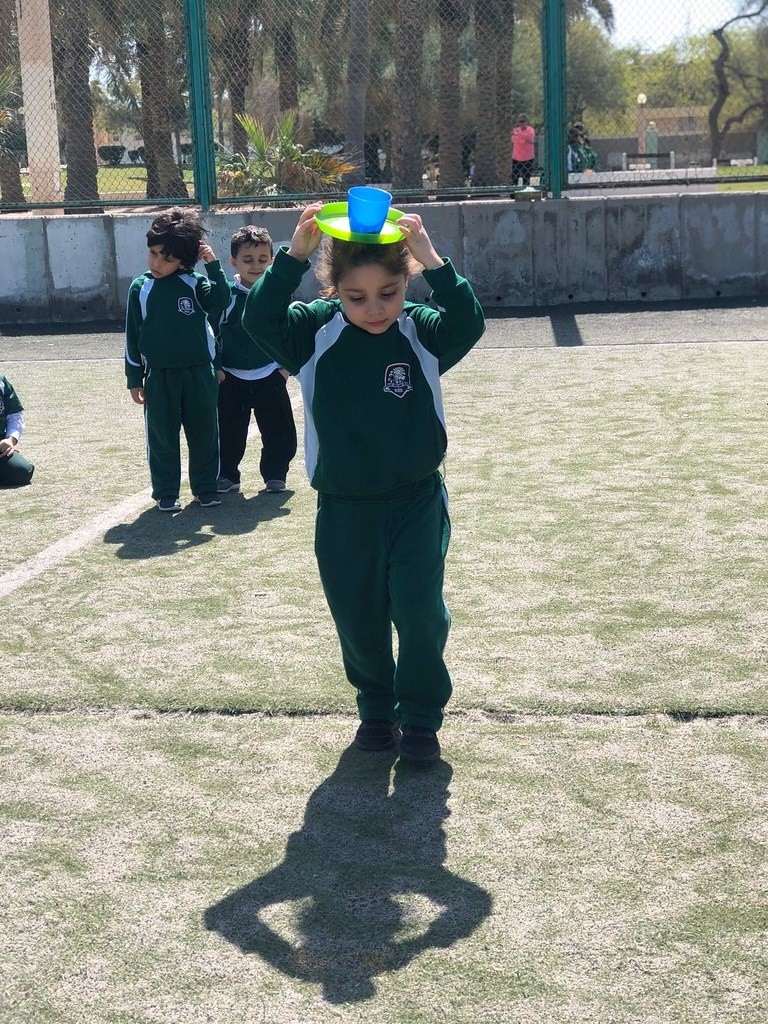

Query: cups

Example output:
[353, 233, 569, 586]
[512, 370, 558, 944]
[347, 187, 393, 235]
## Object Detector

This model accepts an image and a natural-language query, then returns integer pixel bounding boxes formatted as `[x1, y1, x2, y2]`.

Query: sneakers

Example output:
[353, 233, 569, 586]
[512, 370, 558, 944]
[354, 716, 396, 750]
[216, 476, 240, 493]
[158, 497, 182, 511]
[194, 493, 221, 508]
[399, 722, 440, 762]
[266, 479, 286, 493]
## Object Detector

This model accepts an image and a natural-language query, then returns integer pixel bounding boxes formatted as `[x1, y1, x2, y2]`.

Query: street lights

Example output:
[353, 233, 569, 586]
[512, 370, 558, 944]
[631, 92, 653, 170]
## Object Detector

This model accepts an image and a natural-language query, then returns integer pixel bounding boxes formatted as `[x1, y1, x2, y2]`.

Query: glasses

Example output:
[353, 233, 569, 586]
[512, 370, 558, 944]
[519, 120, 527, 123]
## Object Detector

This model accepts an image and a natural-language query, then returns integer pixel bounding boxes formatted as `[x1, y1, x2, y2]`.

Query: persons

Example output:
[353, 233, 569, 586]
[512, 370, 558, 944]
[0, 374, 35, 485]
[538, 125, 545, 186]
[125, 208, 232, 511]
[242, 200, 486, 760]
[217, 225, 297, 491]
[510, 113, 535, 199]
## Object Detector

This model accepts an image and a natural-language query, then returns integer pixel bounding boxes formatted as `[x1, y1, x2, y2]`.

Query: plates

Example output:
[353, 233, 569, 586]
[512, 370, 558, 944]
[312, 201, 411, 245]
